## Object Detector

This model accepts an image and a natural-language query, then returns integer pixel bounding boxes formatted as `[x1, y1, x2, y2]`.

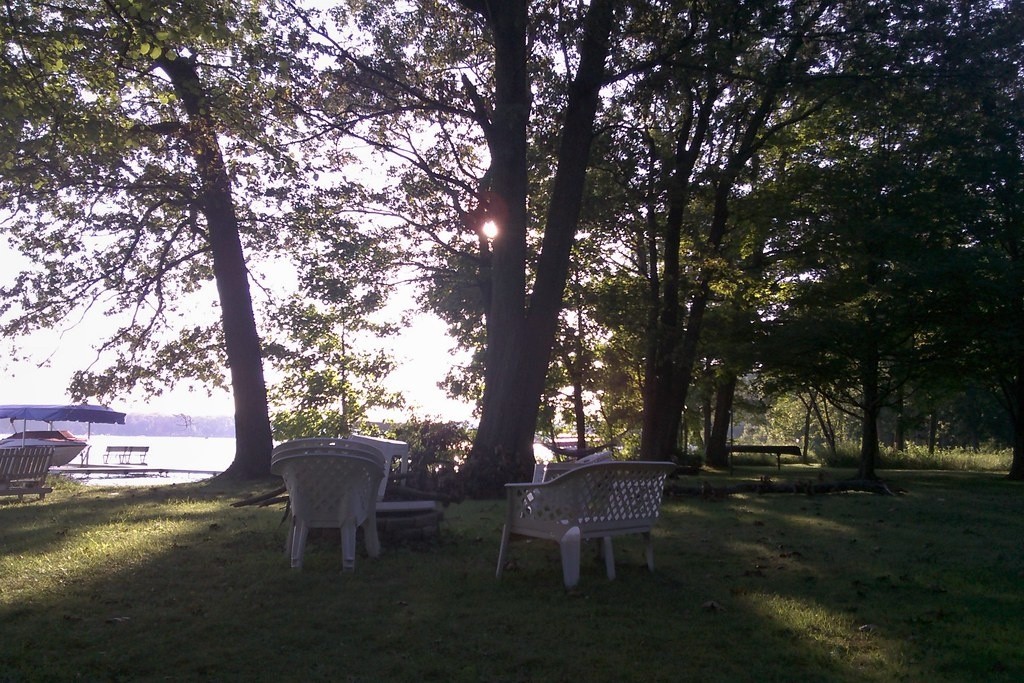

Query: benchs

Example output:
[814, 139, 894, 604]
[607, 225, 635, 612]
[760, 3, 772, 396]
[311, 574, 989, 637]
[0, 446, 56, 499]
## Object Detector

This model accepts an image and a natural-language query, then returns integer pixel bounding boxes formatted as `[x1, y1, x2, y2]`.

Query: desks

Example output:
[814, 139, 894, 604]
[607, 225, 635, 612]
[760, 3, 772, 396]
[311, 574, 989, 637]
[371, 500, 439, 540]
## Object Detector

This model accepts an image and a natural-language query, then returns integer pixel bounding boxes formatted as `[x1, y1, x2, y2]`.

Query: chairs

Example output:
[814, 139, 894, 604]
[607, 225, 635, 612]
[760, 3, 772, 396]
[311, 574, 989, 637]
[497, 450, 677, 594]
[271, 435, 385, 569]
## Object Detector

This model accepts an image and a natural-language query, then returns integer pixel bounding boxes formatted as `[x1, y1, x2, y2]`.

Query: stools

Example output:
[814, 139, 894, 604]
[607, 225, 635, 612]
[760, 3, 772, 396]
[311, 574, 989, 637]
[349, 433, 408, 500]
